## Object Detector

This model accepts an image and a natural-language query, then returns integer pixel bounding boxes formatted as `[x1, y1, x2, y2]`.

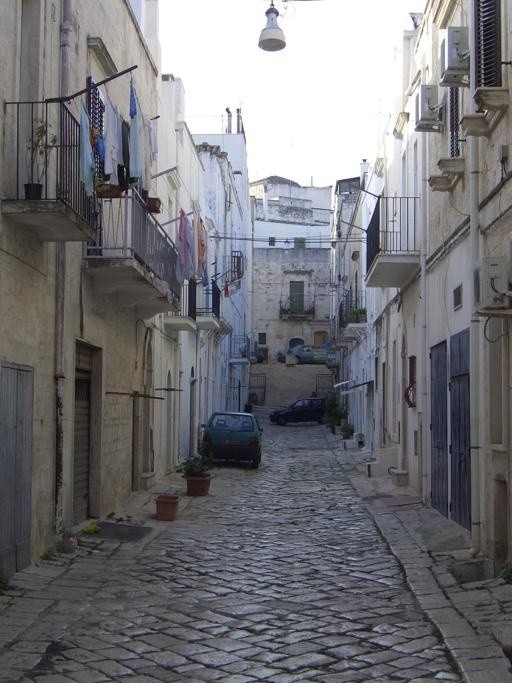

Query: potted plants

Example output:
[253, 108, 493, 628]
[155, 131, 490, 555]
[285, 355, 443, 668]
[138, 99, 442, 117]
[181, 455, 214, 496]
[24, 119, 56, 200]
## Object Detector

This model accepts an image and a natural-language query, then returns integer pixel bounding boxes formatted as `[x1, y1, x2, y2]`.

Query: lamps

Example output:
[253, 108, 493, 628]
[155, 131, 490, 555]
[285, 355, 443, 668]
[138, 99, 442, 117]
[258, 0, 286, 51]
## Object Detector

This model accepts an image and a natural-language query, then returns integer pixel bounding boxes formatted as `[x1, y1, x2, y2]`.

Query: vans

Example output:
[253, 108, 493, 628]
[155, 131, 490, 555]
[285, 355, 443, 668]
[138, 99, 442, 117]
[277, 344, 326, 365]
[270, 397, 328, 426]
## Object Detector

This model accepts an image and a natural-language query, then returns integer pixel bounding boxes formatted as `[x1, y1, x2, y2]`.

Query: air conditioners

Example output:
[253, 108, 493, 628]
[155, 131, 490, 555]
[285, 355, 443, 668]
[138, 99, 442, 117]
[473, 257, 511, 311]
[415, 84, 437, 129]
[438, 27, 470, 86]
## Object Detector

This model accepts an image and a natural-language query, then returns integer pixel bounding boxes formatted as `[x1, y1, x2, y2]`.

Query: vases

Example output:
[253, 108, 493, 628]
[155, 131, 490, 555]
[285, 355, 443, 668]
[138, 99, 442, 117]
[96, 183, 122, 198]
[156, 496, 180, 521]
[143, 198, 160, 209]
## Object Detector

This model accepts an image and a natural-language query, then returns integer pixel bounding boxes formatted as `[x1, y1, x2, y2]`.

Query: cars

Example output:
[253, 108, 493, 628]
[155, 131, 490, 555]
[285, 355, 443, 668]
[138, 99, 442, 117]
[201, 412, 262, 470]
